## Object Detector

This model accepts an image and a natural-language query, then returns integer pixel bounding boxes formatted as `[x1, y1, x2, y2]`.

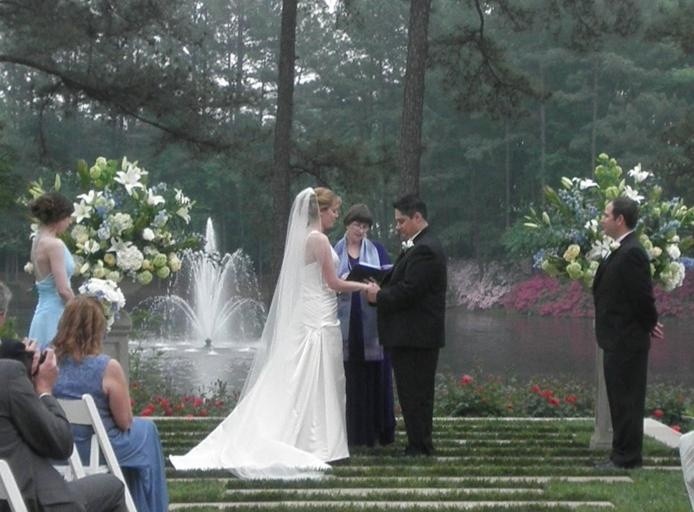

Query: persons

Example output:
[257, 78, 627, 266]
[1, 284, 126, 511]
[40, 294, 167, 512]
[333, 203, 395, 449]
[367, 193, 447, 455]
[26, 193, 75, 353]
[170, 187, 374, 478]
[593, 198, 665, 469]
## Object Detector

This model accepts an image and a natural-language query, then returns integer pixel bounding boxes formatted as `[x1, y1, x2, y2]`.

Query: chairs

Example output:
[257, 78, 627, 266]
[1, 392, 139, 511]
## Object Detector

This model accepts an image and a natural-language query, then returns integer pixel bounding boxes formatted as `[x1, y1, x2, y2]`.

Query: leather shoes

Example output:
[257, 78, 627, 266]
[390, 445, 439, 460]
[594, 457, 646, 471]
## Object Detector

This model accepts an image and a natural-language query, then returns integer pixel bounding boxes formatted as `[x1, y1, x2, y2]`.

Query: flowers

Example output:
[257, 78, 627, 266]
[518, 154, 694, 292]
[402, 239, 414, 252]
[15, 156, 207, 312]
